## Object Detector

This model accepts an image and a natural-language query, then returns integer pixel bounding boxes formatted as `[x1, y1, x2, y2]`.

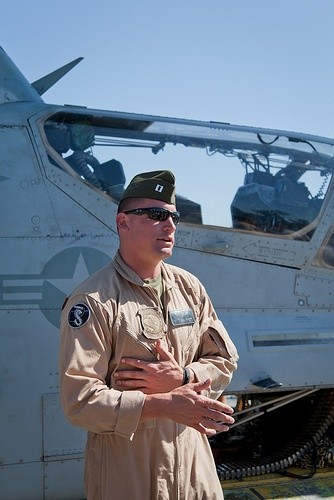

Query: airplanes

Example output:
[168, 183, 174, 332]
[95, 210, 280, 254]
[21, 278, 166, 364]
[1, 42, 334, 498]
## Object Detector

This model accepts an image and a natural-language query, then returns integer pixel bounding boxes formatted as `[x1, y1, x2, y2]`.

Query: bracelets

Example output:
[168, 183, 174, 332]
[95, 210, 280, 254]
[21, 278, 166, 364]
[181, 364, 191, 385]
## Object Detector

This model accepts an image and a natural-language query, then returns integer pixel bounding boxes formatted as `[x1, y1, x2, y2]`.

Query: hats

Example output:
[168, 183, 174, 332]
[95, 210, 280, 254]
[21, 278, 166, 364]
[119, 172, 178, 207]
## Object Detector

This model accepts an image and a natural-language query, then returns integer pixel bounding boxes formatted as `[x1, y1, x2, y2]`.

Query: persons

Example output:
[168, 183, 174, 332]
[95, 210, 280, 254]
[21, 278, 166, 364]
[57, 170, 239, 500]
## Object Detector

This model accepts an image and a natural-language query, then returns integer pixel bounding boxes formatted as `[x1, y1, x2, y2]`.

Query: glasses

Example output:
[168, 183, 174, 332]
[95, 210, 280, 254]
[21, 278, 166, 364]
[122, 207, 180, 225]
